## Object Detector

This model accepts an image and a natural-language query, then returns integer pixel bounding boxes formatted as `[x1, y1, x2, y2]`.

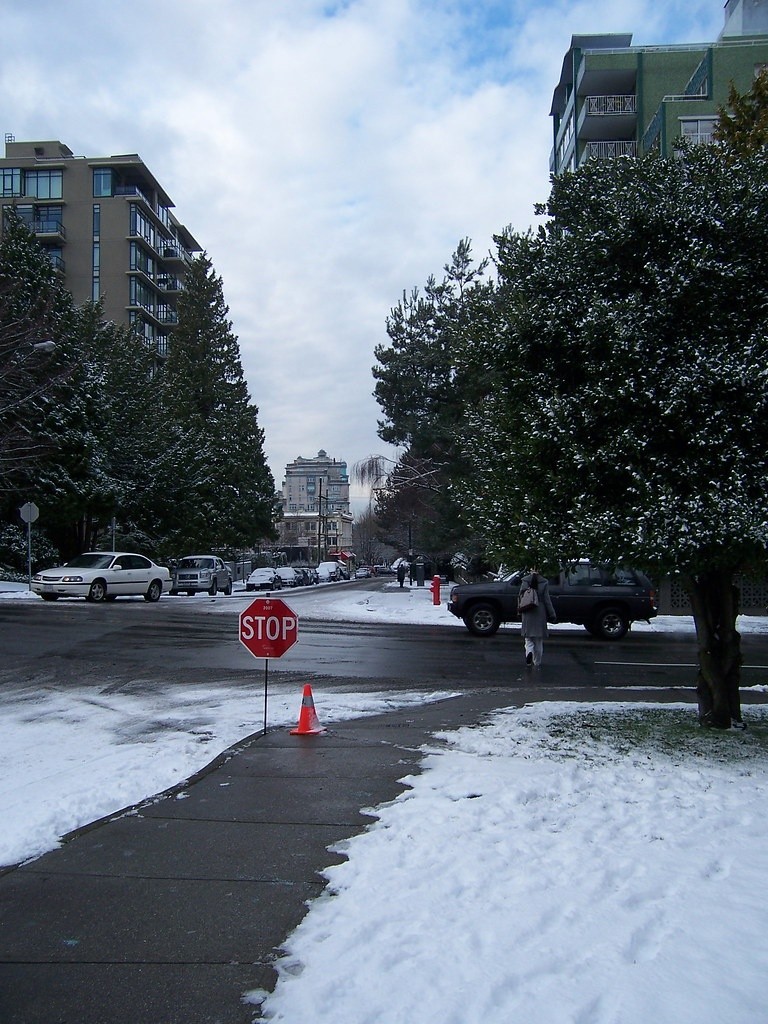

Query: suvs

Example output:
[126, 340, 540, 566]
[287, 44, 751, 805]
[445, 558, 660, 643]
[171, 554, 233, 597]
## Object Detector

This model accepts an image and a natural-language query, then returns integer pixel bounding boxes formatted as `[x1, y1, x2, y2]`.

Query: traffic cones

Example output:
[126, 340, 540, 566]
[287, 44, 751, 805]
[290, 683, 328, 736]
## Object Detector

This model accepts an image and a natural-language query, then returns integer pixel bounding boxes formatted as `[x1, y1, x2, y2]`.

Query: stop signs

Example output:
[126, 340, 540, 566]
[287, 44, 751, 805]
[238, 597, 298, 660]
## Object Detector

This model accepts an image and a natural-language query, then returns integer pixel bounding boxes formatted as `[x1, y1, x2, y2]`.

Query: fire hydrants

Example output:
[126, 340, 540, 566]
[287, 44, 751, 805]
[429, 575, 441, 605]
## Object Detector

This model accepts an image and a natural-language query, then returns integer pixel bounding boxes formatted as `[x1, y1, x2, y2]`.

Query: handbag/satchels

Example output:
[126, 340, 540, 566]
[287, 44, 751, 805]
[519, 576, 538, 612]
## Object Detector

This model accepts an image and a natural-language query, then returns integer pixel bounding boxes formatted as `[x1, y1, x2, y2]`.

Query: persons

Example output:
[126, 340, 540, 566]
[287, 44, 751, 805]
[517, 564, 556, 665]
[397, 564, 406, 587]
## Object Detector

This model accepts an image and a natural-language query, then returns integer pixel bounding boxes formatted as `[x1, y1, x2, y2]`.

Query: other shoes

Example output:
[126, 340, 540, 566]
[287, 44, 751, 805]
[538, 665, 544, 671]
[400, 586, 404, 588]
[526, 652, 532, 664]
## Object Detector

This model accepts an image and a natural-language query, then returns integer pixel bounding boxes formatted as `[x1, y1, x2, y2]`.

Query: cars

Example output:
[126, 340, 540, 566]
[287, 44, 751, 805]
[30, 550, 175, 600]
[355, 568, 369, 579]
[245, 567, 282, 591]
[276, 561, 350, 587]
